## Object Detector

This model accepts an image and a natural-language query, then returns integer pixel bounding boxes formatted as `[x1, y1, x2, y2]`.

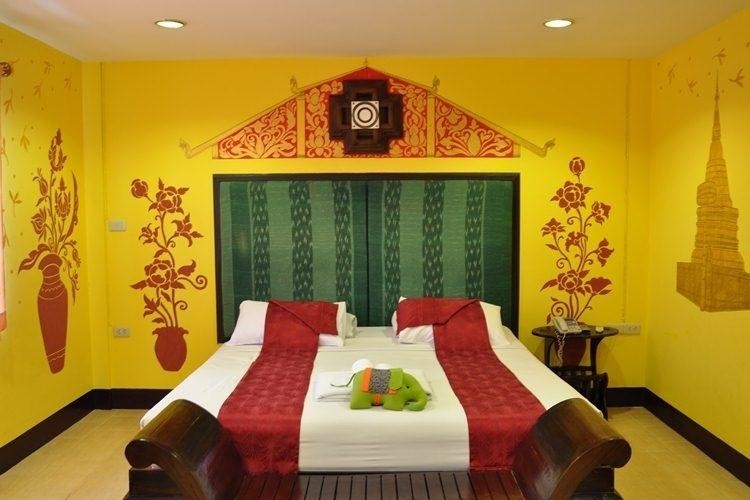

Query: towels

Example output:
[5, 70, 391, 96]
[315, 368, 433, 402]
[374, 362, 388, 370]
[352, 358, 373, 375]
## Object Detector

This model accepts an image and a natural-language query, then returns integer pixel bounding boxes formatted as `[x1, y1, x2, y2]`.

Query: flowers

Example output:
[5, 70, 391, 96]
[122, 169, 209, 328]
[538, 155, 613, 325]
[17, 128, 84, 304]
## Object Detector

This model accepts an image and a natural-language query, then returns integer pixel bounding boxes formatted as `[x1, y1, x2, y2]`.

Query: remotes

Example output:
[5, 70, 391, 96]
[595, 326, 604, 332]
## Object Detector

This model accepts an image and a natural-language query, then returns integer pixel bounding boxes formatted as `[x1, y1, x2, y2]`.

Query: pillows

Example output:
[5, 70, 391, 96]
[345, 310, 358, 338]
[227, 296, 348, 348]
[392, 294, 513, 349]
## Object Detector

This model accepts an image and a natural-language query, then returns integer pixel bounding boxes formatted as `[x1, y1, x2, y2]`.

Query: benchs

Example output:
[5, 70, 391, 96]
[124, 395, 633, 499]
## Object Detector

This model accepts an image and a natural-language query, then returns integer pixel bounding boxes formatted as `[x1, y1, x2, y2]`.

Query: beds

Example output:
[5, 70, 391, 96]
[124, 284, 617, 499]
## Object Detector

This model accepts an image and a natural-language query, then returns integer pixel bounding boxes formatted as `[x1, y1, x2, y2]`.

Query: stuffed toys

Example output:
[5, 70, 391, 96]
[330, 367, 427, 412]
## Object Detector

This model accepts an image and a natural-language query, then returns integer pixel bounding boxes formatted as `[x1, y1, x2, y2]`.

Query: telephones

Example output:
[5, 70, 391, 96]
[551, 316, 582, 334]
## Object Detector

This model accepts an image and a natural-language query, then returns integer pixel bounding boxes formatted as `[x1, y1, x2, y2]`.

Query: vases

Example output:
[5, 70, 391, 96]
[553, 320, 588, 369]
[33, 252, 72, 375]
[151, 323, 190, 373]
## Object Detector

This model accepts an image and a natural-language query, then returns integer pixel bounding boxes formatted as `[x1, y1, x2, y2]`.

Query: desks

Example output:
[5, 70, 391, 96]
[529, 322, 619, 423]
